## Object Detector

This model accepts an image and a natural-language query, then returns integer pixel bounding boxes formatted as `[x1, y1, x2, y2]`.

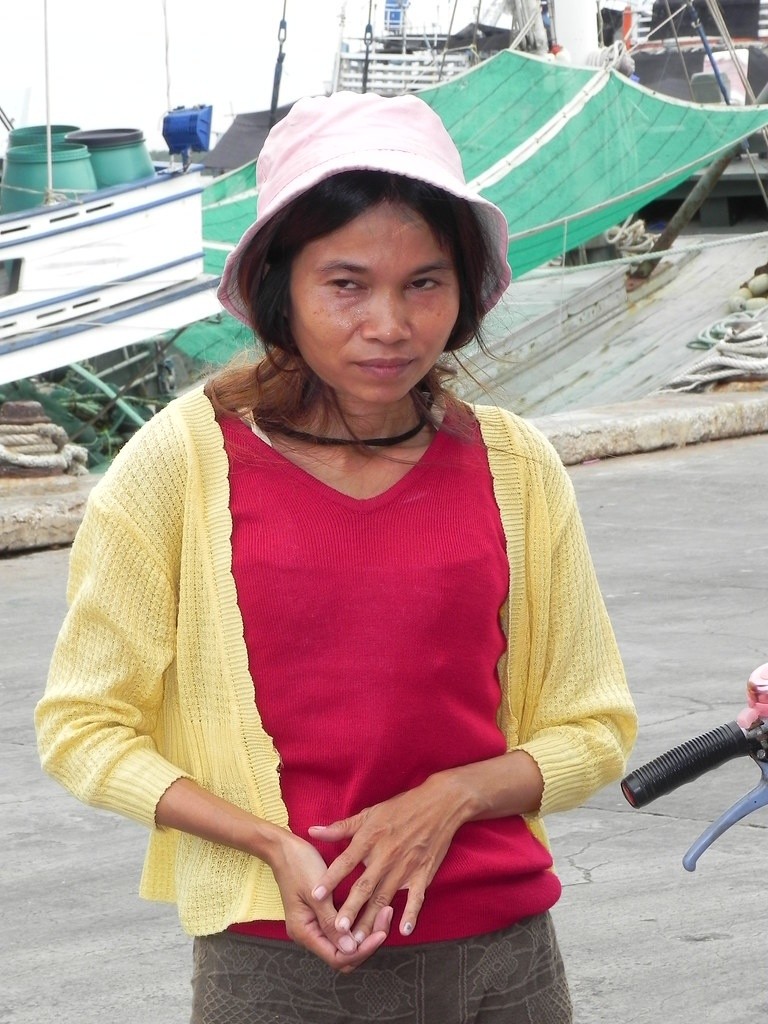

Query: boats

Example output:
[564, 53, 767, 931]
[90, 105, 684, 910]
[0, 159, 226, 390]
[322, 1, 768, 176]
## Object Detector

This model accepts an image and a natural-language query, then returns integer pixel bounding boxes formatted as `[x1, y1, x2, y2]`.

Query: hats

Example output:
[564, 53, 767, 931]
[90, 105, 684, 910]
[216, 91, 512, 352]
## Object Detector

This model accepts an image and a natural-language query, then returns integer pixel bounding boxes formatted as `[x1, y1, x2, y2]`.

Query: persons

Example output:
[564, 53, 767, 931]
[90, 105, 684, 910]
[33, 90, 638, 1024]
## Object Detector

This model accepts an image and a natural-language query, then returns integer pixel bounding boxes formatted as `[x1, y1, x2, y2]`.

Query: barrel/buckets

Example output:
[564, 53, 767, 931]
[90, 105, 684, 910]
[65, 128, 155, 190]
[9, 124, 80, 148]
[0, 143, 98, 279]
